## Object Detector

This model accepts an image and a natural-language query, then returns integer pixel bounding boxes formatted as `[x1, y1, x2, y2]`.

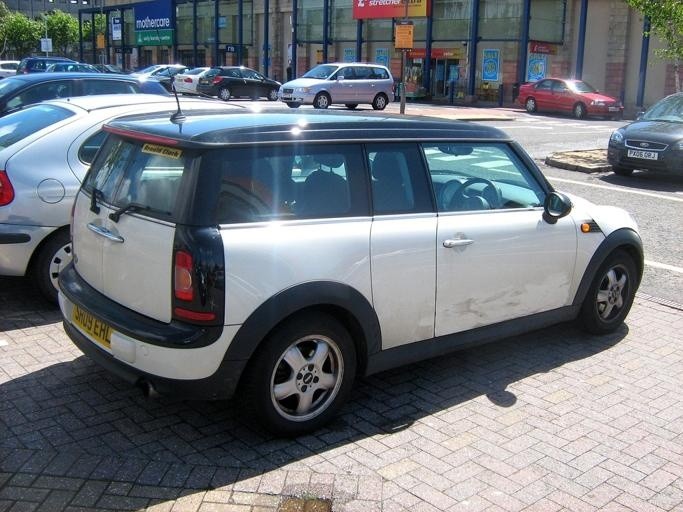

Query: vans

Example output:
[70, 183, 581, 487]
[278, 62, 396, 109]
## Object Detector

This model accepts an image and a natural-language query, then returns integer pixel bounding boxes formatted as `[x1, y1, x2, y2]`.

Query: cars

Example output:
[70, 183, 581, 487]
[606, 91, 682, 178]
[0, 56, 210, 95]
[59, 109, 644, 439]
[0, 72, 164, 111]
[518, 78, 623, 120]
[198, 66, 281, 101]
[0, 94, 248, 302]
